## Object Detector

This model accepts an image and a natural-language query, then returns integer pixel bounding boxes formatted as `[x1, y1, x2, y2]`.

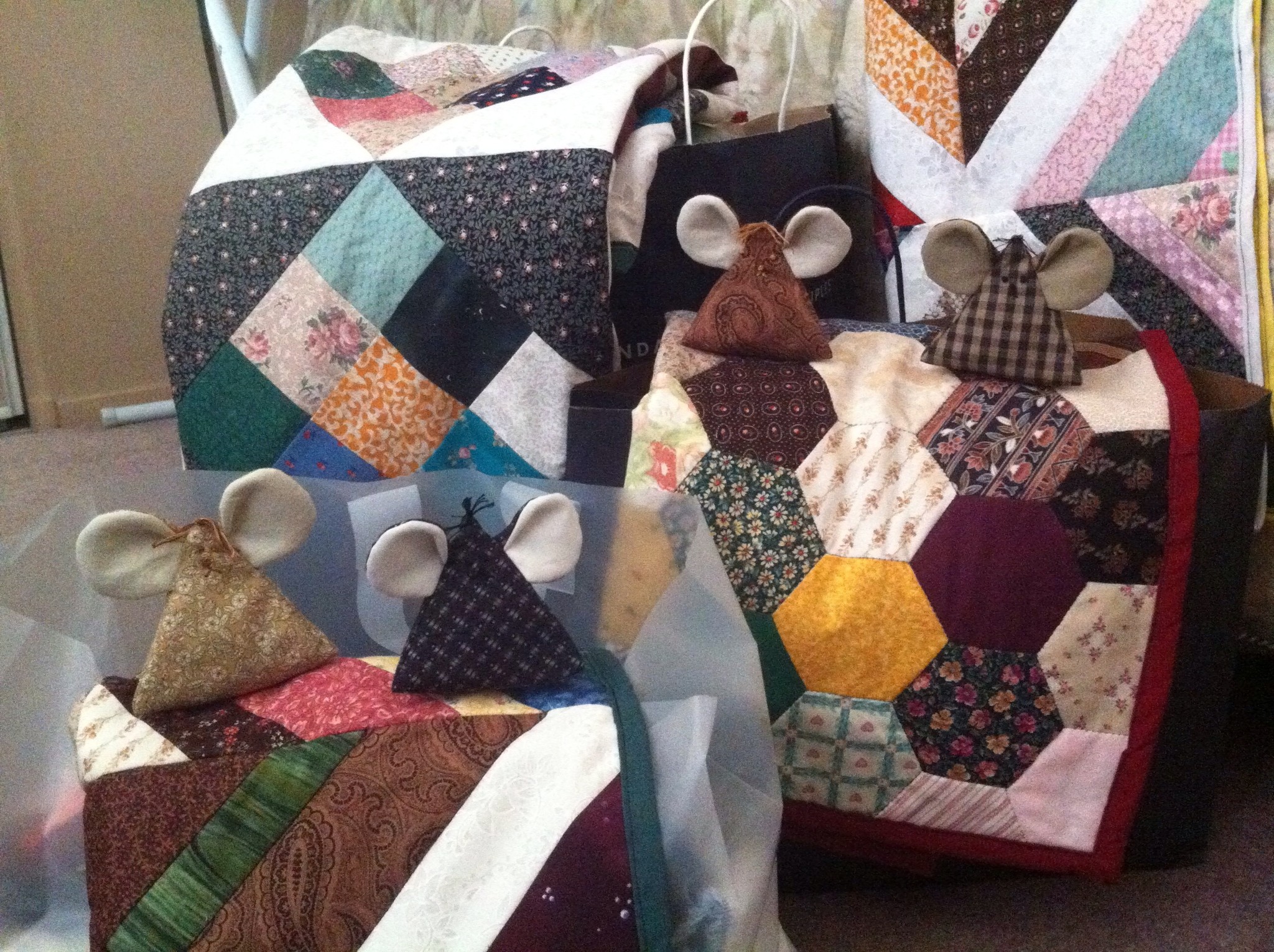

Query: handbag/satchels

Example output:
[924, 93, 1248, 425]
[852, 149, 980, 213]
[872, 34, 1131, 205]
[544, 186, 1272, 874]
[500, 0, 887, 373]
